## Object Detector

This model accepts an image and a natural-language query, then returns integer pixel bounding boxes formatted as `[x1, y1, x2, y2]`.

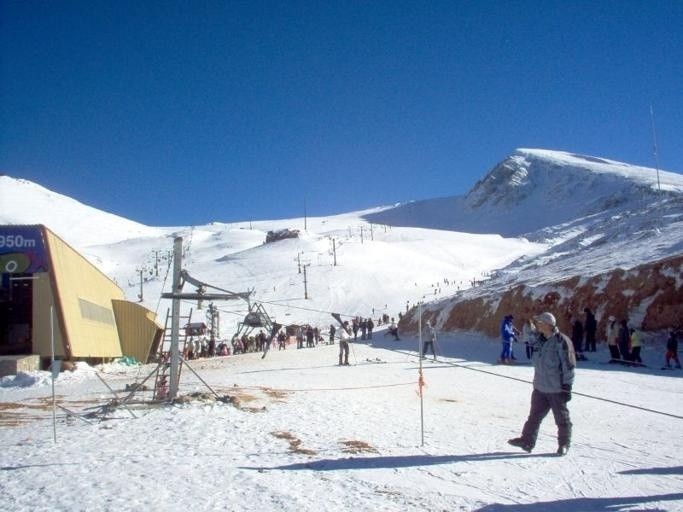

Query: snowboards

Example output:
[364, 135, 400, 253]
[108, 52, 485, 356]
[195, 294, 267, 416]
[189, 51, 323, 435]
[608, 359, 646, 367]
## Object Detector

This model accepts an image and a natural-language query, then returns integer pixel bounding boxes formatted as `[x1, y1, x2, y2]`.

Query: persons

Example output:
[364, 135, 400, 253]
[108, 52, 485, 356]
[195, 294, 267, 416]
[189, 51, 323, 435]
[421, 319, 438, 361]
[661, 330, 682, 368]
[507, 310, 574, 455]
[338, 320, 351, 367]
[497, 308, 642, 367]
[185, 311, 403, 361]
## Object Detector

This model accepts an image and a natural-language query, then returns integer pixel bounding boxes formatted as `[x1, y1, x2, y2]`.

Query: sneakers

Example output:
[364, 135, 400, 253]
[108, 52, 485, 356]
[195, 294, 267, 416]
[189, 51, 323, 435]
[508, 438, 535, 452]
[558, 444, 568, 455]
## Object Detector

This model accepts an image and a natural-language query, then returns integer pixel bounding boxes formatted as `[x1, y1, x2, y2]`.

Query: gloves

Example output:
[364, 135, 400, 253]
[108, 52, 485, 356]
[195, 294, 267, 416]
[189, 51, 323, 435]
[561, 384, 572, 401]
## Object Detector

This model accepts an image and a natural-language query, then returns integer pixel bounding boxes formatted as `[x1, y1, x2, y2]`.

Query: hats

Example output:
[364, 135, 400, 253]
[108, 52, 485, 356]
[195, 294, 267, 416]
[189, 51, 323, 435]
[534, 312, 556, 326]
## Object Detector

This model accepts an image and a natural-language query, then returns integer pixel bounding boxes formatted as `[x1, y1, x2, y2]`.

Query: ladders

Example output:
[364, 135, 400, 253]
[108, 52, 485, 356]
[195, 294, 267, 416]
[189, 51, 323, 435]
[153, 306, 194, 402]
